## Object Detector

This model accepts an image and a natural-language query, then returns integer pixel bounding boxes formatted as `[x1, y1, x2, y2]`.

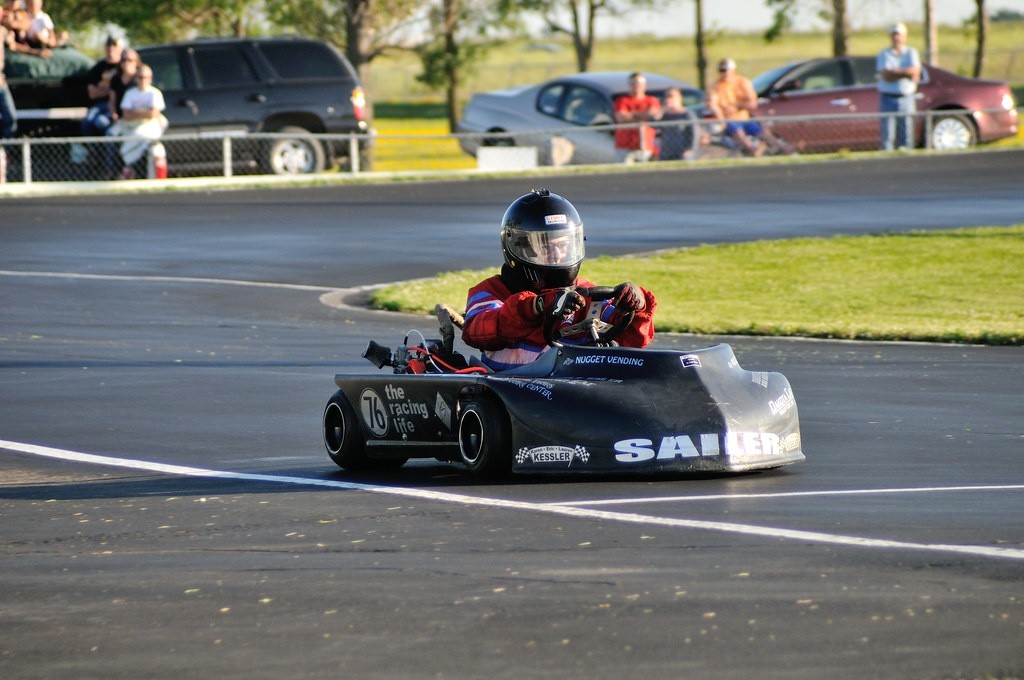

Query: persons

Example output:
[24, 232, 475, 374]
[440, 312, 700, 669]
[462, 187, 657, 373]
[614, 59, 806, 162]
[876, 22, 922, 149]
[0, 0, 68, 138]
[84, 36, 166, 180]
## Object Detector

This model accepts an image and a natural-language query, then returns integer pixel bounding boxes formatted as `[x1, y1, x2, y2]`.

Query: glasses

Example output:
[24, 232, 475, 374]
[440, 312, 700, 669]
[138, 75, 151, 80]
[719, 66, 727, 72]
[541, 240, 571, 255]
[107, 41, 117, 46]
[121, 58, 134, 62]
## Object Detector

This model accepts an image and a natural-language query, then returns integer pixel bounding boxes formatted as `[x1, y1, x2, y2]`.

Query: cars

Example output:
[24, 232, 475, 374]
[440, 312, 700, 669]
[701, 54, 1016, 153]
[458, 71, 796, 166]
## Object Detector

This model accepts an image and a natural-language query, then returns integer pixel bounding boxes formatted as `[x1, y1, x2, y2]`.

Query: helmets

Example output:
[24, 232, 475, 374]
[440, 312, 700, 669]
[500, 187, 587, 288]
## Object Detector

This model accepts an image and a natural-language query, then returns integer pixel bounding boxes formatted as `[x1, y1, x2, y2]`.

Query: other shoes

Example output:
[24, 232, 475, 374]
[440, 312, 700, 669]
[752, 141, 767, 157]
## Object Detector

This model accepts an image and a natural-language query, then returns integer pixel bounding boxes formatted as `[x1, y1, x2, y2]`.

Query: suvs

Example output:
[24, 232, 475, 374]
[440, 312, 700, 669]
[13, 34, 374, 181]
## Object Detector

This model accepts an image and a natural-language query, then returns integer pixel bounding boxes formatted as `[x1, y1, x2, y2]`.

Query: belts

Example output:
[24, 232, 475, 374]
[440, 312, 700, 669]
[882, 94, 905, 98]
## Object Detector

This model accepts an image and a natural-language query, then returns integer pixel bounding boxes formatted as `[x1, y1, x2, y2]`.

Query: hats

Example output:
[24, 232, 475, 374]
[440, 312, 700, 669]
[890, 23, 907, 35]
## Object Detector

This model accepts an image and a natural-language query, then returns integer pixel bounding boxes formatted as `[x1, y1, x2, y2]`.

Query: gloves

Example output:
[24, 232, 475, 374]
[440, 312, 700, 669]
[612, 281, 644, 317]
[536, 290, 586, 321]
